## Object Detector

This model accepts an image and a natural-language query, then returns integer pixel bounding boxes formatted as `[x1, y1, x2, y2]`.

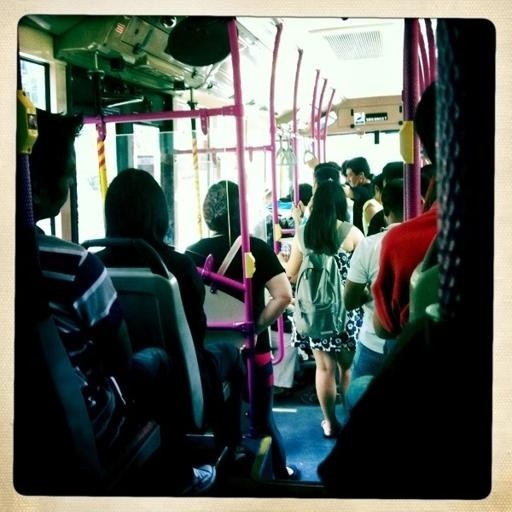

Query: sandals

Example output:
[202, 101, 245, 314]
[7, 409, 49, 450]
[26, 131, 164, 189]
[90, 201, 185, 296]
[319, 419, 340, 437]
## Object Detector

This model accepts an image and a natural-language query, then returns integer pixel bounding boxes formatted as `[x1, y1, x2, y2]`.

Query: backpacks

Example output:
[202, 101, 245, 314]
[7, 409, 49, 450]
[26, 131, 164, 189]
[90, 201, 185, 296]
[293, 219, 354, 341]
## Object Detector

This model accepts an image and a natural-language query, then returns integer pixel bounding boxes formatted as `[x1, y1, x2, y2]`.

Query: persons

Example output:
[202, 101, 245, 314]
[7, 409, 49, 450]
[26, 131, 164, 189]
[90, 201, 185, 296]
[177, 179, 300, 484]
[282, 178, 368, 440]
[83, 168, 230, 446]
[282, 155, 441, 405]
[22, 107, 217, 496]
[370, 79, 438, 354]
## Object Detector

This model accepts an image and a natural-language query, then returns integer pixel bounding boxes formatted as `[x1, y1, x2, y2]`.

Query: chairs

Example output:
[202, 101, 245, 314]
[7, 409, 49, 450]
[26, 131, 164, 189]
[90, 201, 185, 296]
[80, 239, 231, 439]
[17, 236, 106, 495]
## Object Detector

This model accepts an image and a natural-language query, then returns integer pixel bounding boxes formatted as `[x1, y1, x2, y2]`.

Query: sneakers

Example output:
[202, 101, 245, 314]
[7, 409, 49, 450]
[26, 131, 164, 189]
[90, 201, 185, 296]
[193, 463, 218, 494]
[287, 464, 299, 478]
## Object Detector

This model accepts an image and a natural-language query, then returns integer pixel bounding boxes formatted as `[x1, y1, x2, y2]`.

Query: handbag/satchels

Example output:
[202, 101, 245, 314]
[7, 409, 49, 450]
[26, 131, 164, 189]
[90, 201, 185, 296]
[202, 282, 249, 351]
